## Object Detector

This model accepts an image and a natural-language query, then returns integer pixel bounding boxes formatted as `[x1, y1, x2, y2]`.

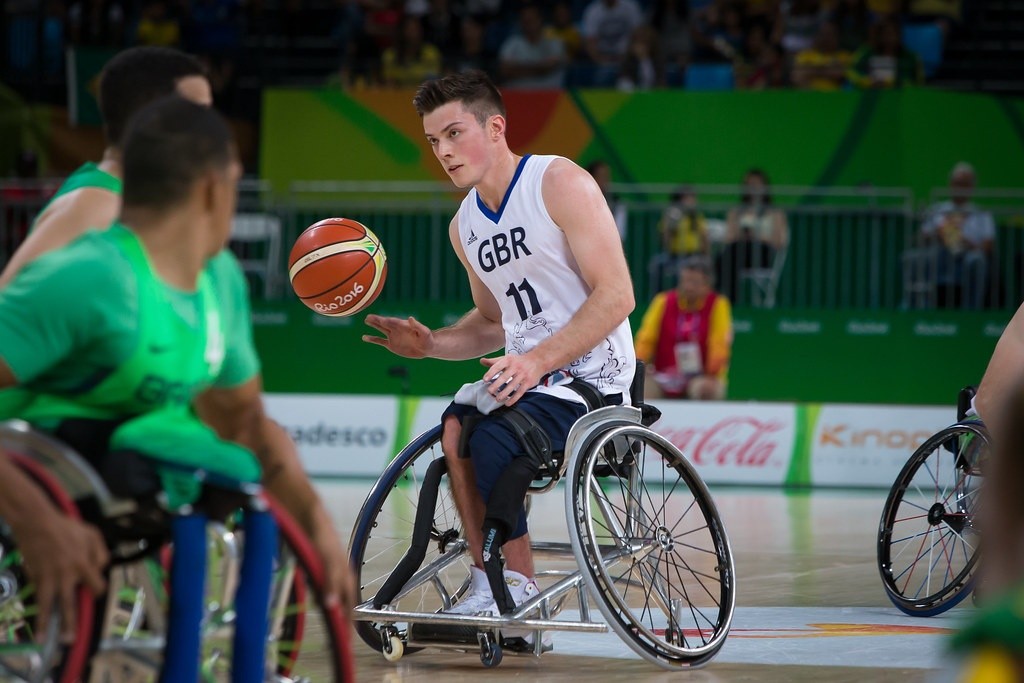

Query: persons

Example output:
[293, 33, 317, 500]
[964, 288, 1024, 683]
[0, 44, 361, 680]
[584, 158, 790, 312]
[921, 158, 998, 313]
[635, 253, 735, 402]
[363, 70, 639, 654]
[461, 0, 1019, 92]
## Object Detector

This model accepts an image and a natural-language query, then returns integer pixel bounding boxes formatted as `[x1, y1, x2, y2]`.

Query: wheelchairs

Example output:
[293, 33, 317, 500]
[876, 387, 991, 615]
[0, 416, 360, 683]
[339, 358, 738, 669]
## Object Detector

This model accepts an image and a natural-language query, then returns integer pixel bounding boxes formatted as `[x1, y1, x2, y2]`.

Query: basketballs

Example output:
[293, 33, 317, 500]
[288, 218, 391, 319]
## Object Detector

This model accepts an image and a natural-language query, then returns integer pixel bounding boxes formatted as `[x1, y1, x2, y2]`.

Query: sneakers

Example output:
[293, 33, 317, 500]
[463, 569, 553, 652]
[411, 564, 495, 640]
